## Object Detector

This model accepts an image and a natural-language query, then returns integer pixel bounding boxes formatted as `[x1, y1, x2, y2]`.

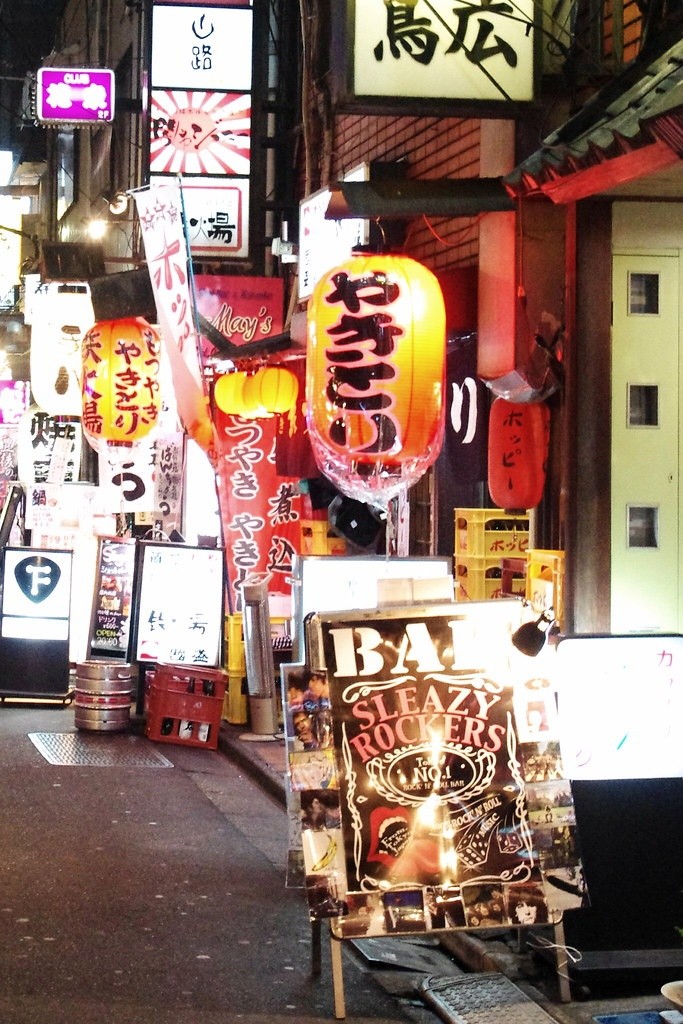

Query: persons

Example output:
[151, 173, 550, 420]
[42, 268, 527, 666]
[513, 687, 579, 865]
[309, 866, 585, 937]
[284, 665, 340, 829]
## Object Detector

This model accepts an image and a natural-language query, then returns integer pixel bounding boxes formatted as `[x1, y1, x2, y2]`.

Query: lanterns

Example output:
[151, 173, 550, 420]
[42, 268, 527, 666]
[488, 387, 550, 516]
[305, 252, 447, 503]
[214, 362, 299, 422]
[80, 316, 160, 544]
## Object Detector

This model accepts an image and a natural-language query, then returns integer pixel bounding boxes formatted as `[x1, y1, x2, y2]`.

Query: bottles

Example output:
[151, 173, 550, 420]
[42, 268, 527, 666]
[197, 680, 214, 741]
[179, 677, 194, 739]
[161, 717, 173, 736]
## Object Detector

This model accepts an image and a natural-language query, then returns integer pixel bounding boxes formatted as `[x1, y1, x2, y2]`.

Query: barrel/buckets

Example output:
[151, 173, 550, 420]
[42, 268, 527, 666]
[77, 660, 131, 729]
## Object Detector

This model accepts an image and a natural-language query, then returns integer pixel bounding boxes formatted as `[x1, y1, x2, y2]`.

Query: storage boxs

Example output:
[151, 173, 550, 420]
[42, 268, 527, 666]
[144, 614, 292, 749]
[454, 508, 566, 632]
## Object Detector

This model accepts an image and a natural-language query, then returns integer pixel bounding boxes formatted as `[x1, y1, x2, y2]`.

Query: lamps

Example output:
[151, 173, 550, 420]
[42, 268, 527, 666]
[511, 598, 558, 657]
[99, 184, 150, 214]
